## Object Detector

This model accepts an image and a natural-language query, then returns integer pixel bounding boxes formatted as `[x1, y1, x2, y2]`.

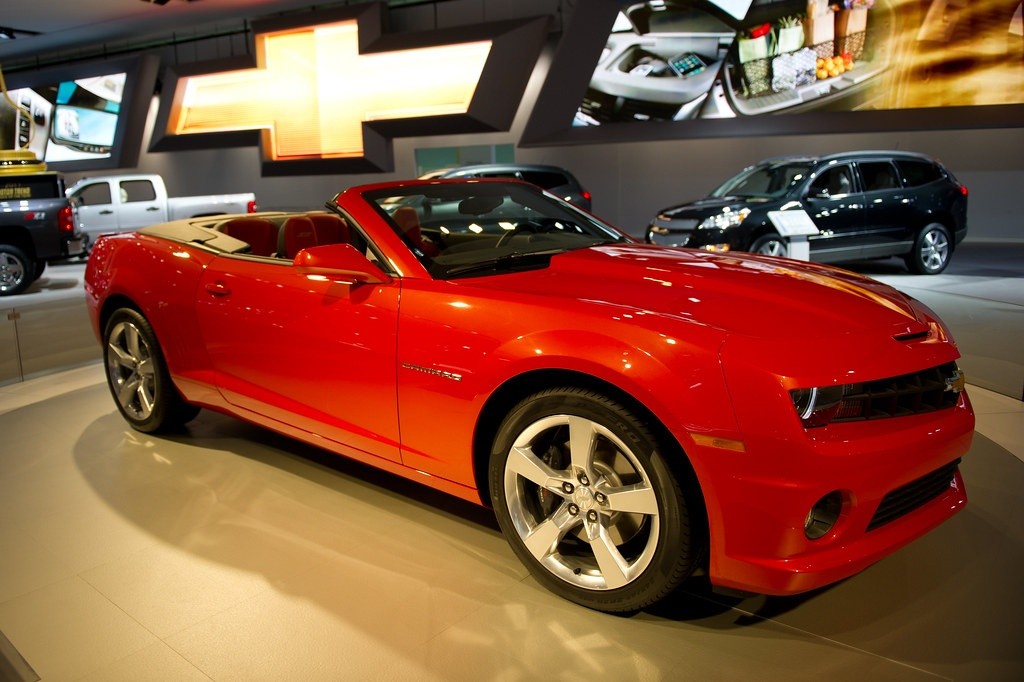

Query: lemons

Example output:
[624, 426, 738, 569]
[816, 55, 855, 79]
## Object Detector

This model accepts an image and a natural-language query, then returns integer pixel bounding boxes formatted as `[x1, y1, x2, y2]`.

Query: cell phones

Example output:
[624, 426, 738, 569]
[668, 51, 708, 76]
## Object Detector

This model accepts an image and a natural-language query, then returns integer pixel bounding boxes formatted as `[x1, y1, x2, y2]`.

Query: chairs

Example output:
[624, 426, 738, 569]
[224, 207, 441, 261]
[868, 164, 898, 190]
[828, 166, 853, 195]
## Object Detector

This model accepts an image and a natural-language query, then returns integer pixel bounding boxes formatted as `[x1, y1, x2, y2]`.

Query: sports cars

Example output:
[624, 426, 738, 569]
[84, 179, 976, 614]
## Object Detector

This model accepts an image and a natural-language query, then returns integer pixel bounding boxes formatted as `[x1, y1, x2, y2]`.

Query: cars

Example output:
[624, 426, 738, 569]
[378, 163, 591, 230]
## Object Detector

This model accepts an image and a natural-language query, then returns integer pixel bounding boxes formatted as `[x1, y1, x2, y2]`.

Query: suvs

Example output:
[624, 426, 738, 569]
[644, 149, 969, 276]
[0, 174, 85, 297]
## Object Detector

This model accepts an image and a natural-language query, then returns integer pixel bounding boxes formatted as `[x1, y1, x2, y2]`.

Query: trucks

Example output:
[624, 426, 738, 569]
[47, 174, 257, 265]
